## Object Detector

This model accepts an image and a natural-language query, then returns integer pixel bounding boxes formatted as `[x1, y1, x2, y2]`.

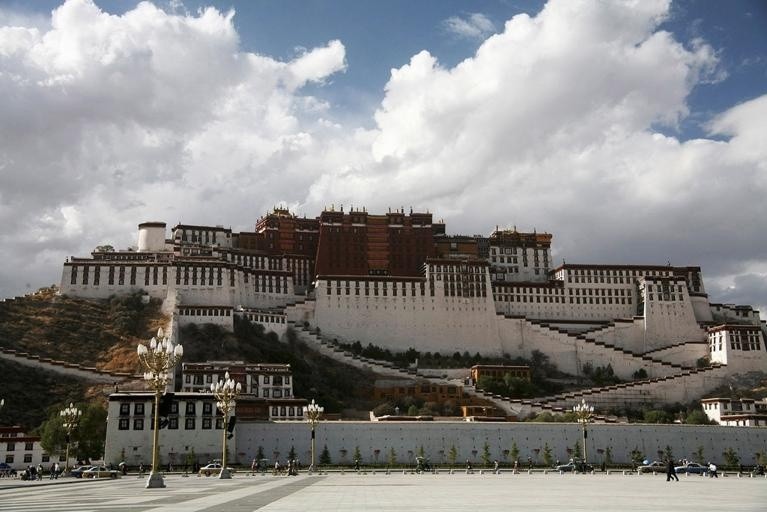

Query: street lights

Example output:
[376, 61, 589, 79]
[0, 398, 6, 410]
[133, 325, 186, 489]
[572, 397, 597, 463]
[301, 397, 325, 472]
[59, 400, 85, 478]
[208, 371, 244, 479]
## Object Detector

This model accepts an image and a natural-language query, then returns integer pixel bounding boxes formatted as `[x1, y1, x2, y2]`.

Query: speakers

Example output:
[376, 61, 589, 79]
[228, 434, 233, 440]
[228, 416, 236, 432]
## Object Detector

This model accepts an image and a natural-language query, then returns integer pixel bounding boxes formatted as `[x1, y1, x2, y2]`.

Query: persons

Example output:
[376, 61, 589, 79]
[666, 456, 679, 481]
[118, 456, 299, 477]
[20, 463, 60, 482]
[664, 458, 674, 479]
[493, 458, 498, 473]
[466, 459, 472, 473]
[353, 459, 360, 470]
[554, 456, 719, 478]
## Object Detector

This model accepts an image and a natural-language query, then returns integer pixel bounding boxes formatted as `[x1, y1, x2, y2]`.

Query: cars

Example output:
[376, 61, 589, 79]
[198, 463, 235, 477]
[672, 461, 708, 474]
[81, 465, 123, 479]
[556, 461, 595, 473]
[638, 461, 667, 473]
[70, 464, 93, 478]
